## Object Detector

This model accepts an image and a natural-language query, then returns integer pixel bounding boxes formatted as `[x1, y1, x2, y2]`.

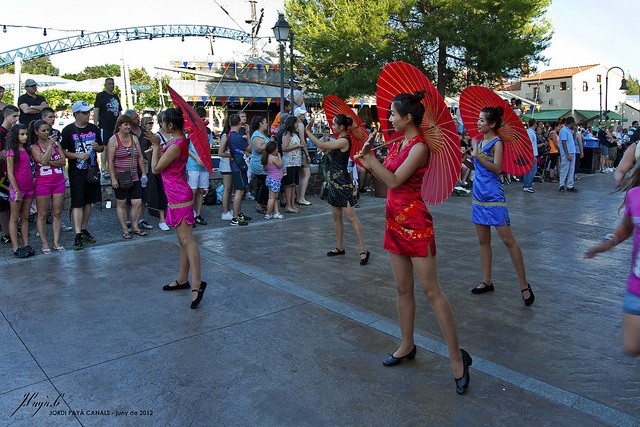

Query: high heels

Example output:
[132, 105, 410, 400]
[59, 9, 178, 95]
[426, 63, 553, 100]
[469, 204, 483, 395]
[383, 344, 416, 366]
[453, 348, 473, 394]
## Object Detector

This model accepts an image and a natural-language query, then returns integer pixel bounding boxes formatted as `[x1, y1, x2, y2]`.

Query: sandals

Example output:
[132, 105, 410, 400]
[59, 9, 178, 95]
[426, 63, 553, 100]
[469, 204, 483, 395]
[190, 281, 207, 308]
[521, 283, 535, 306]
[359, 250, 370, 265]
[471, 281, 495, 294]
[327, 247, 346, 257]
[162, 279, 190, 291]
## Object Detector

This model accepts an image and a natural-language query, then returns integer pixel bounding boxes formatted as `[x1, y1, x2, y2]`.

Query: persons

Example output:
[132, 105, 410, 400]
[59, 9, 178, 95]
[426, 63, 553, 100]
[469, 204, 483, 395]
[499, 97, 598, 194]
[456, 107, 466, 135]
[362, 125, 475, 197]
[306, 113, 371, 266]
[467, 105, 536, 308]
[583, 160, 640, 355]
[191, 99, 335, 226]
[142, 106, 208, 310]
[613, 140, 640, 184]
[347, 158, 368, 198]
[598, 121, 622, 174]
[630, 127, 640, 143]
[622, 128, 630, 152]
[0, 74, 170, 258]
[628, 121, 638, 138]
[354, 88, 472, 398]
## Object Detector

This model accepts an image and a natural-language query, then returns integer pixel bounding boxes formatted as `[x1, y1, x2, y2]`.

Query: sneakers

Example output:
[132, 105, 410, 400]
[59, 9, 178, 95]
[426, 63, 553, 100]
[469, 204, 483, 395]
[13, 248, 29, 258]
[22, 245, 36, 255]
[237, 213, 252, 221]
[81, 229, 96, 243]
[159, 222, 169, 230]
[72, 233, 84, 249]
[230, 217, 248, 226]
[195, 214, 208, 225]
[221, 209, 234, 220]
[138, 220, 155, 230]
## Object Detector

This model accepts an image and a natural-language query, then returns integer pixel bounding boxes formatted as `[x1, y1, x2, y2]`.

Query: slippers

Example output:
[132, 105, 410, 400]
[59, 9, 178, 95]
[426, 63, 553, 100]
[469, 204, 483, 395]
[120, 232, 133, 240]
[131, 228, 147, 238]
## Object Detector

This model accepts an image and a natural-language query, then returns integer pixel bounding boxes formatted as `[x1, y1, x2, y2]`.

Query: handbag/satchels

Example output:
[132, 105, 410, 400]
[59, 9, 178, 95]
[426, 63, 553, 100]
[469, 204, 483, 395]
[114, 133, 135, 189]
[73, 121, 101, 182]
[227, 131, 248, 172]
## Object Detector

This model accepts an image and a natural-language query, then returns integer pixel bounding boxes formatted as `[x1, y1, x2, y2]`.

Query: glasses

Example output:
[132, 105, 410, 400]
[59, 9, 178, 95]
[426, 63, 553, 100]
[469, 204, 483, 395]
[294, 107, 308, 117]
[125, 145, 131, 157]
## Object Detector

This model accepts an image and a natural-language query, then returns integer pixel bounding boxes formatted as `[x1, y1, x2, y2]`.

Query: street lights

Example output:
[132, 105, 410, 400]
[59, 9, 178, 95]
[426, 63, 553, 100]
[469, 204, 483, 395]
[271, 12, 293, 117]
[605, 66, 630, 123]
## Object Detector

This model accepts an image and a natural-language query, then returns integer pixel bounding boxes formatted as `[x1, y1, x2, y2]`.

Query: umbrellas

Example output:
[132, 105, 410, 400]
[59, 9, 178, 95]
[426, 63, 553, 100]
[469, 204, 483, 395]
[144, 84, 215, 182]
[459, 86, 534, 177]
[353, 61, 462, 206]
[317, 95, 378, 174]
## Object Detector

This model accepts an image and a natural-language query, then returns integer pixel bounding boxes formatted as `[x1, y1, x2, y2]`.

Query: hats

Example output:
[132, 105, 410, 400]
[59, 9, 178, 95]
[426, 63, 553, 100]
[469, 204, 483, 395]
[71, 100, 94, 114]
[24, 79, 37, 87]
